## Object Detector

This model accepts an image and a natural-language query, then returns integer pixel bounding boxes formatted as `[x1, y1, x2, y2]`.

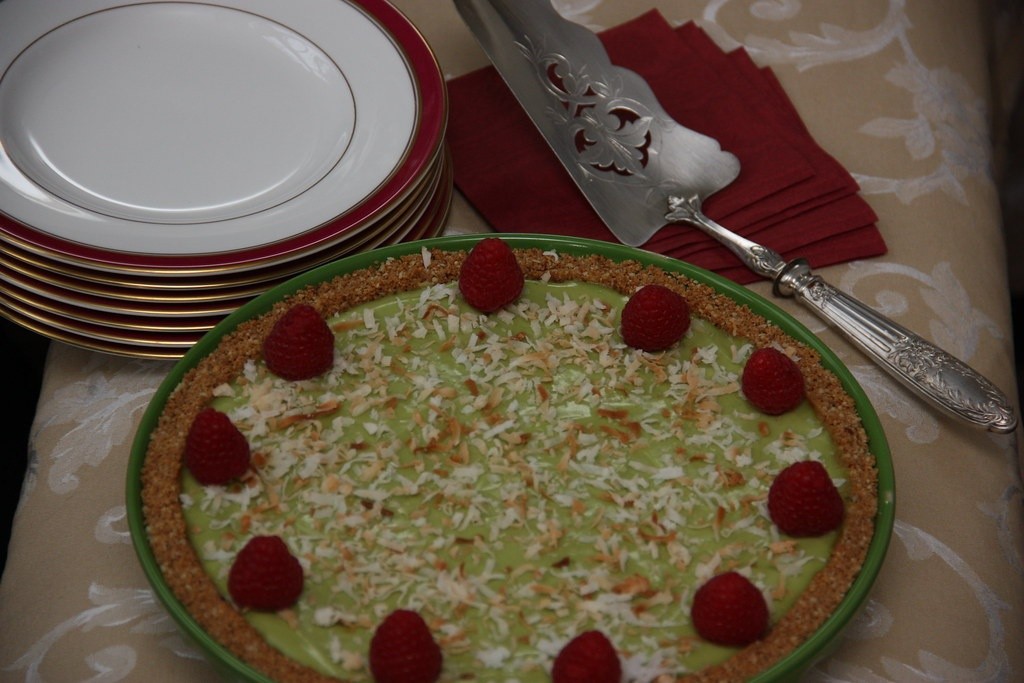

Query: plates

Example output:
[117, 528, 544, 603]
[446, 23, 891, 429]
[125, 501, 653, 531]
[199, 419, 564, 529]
[127, 232, 896, 683]
[0, 0, 452, 362]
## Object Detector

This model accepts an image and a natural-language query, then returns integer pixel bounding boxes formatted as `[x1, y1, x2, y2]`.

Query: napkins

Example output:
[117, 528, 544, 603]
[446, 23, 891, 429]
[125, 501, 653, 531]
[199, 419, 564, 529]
[443, 8, 887, 285]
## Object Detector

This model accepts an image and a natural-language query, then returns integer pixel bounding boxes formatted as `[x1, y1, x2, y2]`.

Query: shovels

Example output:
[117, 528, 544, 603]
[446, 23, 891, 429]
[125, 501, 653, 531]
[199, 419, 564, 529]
[452, 0, 1018, 434]
